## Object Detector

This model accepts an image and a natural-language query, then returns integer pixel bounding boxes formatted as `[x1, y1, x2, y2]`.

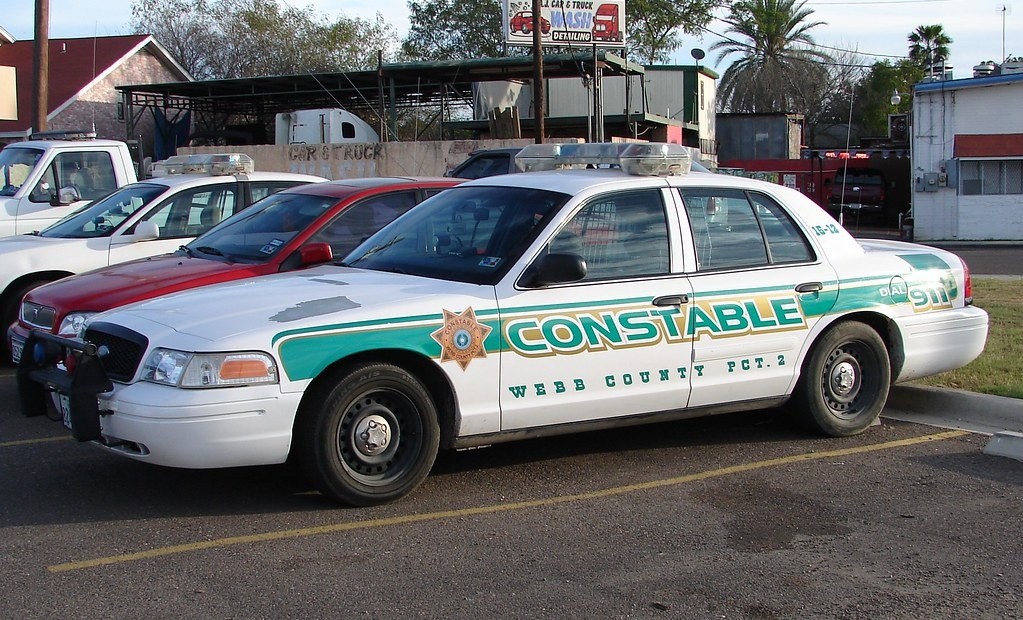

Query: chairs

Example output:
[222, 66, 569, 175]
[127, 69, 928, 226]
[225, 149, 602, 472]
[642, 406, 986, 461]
[198, 206, 221, 235]
[622, 231, 665, 274]
[319, 203, 372, 252]
[551, 231, 582, 255]
[69, 166, 96, 199]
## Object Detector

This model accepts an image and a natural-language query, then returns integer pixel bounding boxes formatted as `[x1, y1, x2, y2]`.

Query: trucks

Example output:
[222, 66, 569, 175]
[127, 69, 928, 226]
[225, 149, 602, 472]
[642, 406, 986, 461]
[0, 139, 139, 237]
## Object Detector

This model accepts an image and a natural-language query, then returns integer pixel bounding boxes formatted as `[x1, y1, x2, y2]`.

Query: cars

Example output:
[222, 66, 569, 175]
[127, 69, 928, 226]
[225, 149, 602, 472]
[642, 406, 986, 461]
[0, 154, 333, 345]
[444, 149, 787, 262]
[16, 143, 990, 504]
[7, 177, 474, 407]
[829, 167, 905, 226]
